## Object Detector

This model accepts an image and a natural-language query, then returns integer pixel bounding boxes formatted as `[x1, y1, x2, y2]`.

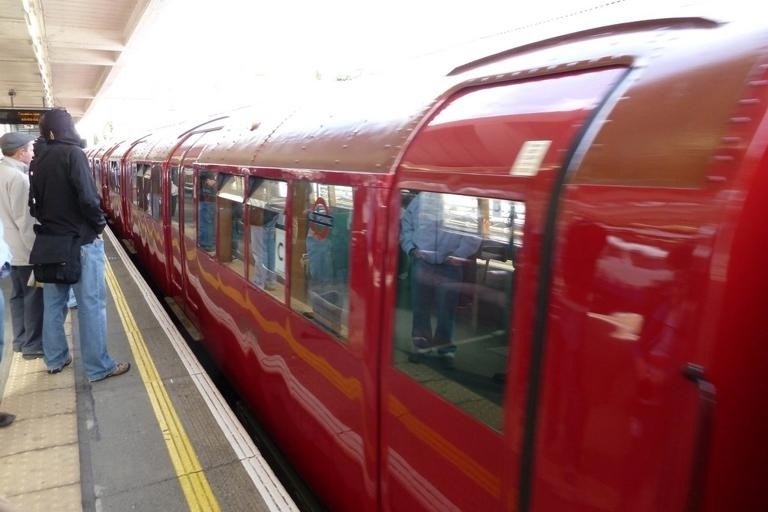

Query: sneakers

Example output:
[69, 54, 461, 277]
[48, 356, 73, 373]
[414, 344, 458, 357]
[106, 363, 131, 377]
[23, 354, 44, 360]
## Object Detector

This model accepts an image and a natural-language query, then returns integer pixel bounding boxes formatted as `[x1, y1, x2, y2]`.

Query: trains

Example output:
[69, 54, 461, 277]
[84, 12, 768, 511]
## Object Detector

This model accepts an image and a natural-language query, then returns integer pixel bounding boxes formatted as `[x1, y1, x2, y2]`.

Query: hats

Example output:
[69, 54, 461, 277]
[1, 133, 35, 151]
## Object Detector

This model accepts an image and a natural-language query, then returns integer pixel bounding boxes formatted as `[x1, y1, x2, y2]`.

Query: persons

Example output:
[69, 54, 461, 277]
[400, 191, 484, 359]
[28, 109, 130, 382]
[0, 131, 43, 360]
[249, 177, 279, 292]
[199, 172, 240, 261]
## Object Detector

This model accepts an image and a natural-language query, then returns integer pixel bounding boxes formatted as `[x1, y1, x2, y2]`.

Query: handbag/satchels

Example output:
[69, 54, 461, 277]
[29, 229, 81, 285]
